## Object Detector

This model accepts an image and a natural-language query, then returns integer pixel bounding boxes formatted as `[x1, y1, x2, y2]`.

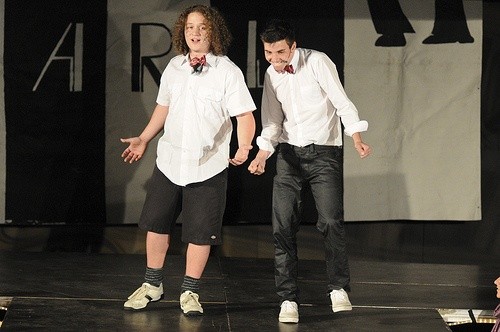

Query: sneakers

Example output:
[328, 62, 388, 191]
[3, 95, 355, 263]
[279, 300, 299, 323]
[180, 290, 203, 315]
[124, 282, 164, 310]
[330, 289, 352, 312]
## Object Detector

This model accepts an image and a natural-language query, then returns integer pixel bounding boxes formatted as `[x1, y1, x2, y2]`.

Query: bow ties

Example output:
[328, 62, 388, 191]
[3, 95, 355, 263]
[190, 55, 207, 73]
[284, 64, 294, 74]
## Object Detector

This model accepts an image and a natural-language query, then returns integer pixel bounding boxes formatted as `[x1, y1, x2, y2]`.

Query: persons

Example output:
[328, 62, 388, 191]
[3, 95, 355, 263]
[247, 25, 369, 325]
[120, 5, 257, 316]
[491, 277, 500, 332]
